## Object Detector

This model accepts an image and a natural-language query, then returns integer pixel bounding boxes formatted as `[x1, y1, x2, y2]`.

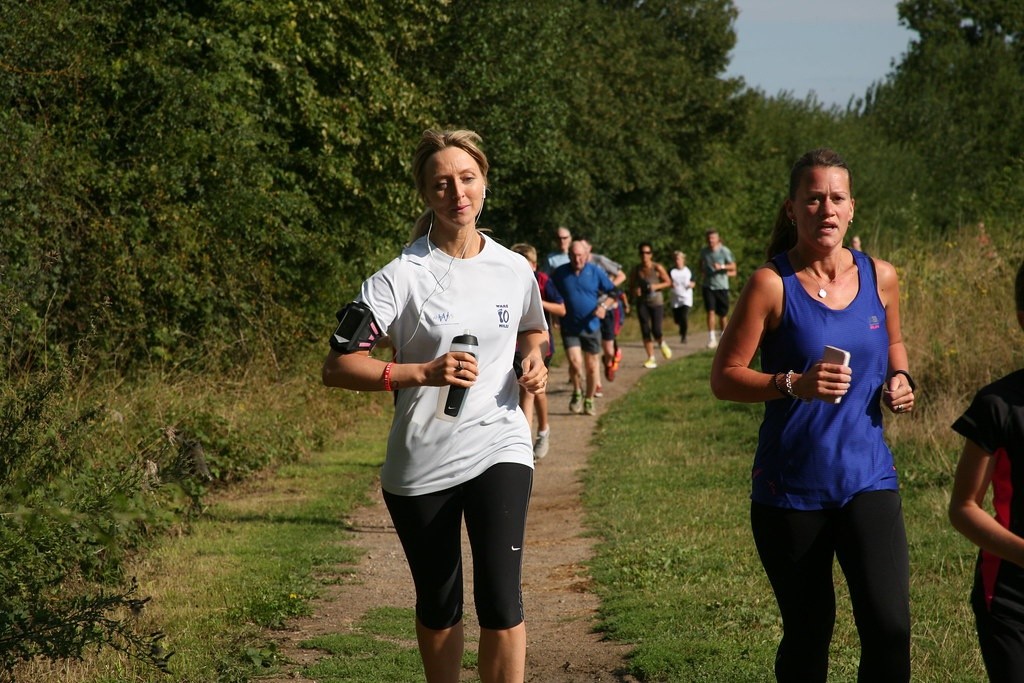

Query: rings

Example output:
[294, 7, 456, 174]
[457, 360, 463, 370]
[898, 404, 903, 411]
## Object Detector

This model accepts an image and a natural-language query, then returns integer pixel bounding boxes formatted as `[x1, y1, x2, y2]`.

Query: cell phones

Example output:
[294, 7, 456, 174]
[330, 303, 371, 350]
[820, 346, 851, 404]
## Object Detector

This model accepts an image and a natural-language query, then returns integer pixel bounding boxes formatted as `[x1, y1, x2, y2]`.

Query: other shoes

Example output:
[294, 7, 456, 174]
[706, 339, 716, 348]
[681, 335, 687, 343]
[569, 377, 573, 384]
[643, 360, 657, 368]
[660, 343, 672, 359]
[594, 385, 603, 397]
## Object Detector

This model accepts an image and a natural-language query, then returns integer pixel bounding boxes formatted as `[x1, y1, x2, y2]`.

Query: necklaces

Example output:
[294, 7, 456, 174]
[799, 251, 840, 298]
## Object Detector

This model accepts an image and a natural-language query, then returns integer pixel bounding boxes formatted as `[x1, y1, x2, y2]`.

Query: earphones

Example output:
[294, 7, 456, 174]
[424, 196, 430, 209]
[483, 185, 487, 199]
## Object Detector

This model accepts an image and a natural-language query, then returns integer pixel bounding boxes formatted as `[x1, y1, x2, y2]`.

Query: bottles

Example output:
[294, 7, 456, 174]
[436, 331, 480, 424]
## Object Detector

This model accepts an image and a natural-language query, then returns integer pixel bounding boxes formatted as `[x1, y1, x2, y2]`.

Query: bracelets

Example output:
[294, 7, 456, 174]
[774, 370, 809, 401]
[600, 302, 608, 309]
[721, 264, 725, 269]
[893, 370, 915, 393]
[384, 362, 392, 392]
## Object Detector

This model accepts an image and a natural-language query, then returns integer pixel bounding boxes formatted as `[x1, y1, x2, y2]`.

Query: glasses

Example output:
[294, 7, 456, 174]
[641, 251, 651, 254]
[558, 237, 568, 240]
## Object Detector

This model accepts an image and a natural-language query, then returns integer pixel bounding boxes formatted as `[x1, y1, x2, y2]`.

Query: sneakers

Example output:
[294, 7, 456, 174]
[570, 388, 584, 413]
[584, 396, 595, 416]
[602, 356, 614, 381]
[612, 351, 622, 371]
[531, 423, 550, 464]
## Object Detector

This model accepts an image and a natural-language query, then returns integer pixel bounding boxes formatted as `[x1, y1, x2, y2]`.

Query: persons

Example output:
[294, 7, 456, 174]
[711, 150, 917, 683]
[511, 243, 566, 458]
[545, 226, 629, 416]
[948, 262, 1024, 683]
[851, 235, 864, 253]
[629, 241, 672, 368]
[667, 251, 696, 344]
[699, 230, 737, 349]
[323, 127, 549, 683]
